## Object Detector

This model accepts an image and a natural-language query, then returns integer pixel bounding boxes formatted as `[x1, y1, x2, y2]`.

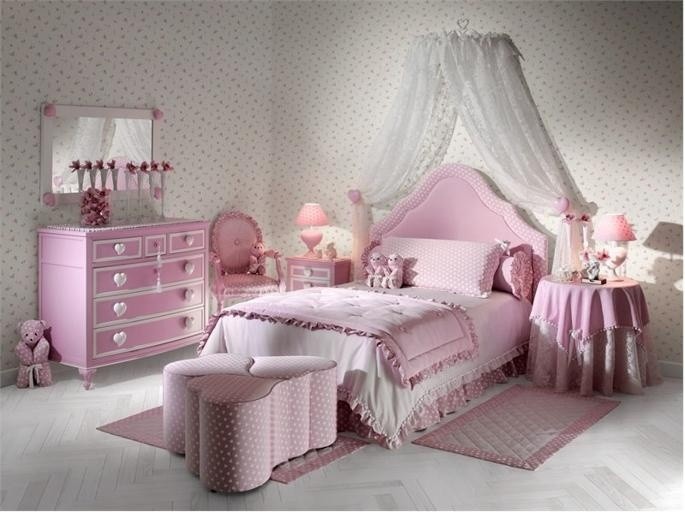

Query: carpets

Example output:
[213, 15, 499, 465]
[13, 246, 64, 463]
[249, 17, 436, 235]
[95, 400, 365, 483]
[410, 383, 623, 470]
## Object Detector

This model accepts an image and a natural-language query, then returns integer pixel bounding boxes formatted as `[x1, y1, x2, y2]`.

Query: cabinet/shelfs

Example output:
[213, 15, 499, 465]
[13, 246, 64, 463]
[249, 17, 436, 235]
[37, 221, 210, 391]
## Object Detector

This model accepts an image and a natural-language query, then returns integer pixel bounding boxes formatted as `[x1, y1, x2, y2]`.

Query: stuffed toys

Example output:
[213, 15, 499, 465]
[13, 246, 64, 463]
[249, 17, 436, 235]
[13, 319, 53, 389]
[382, 254, 404, 289]
[365, 252, 386, 287]
[248, 242, 266, 275]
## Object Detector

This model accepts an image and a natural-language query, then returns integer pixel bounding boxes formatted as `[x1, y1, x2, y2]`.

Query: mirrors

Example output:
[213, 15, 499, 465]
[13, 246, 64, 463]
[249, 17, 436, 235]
[40, 103, 160, 204]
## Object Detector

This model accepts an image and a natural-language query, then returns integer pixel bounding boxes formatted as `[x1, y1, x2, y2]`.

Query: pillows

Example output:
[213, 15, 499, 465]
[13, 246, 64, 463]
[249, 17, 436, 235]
[492, 251, 534, 301]
[361, 239, 380, 279]
[380, 235, 507, 298]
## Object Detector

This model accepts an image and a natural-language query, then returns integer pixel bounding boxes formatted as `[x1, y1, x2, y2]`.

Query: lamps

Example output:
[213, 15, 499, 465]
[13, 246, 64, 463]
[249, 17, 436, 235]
[295, 203, 329, 256]
[590, 213, 636, 282]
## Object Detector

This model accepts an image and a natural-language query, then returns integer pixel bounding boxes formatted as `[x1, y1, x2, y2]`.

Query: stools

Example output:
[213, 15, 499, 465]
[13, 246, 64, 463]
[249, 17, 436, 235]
[161, 353, 338, 493]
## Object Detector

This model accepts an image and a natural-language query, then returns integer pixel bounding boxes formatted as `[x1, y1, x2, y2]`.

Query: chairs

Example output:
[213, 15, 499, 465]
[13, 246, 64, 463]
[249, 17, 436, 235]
[210, 210, 287, 312]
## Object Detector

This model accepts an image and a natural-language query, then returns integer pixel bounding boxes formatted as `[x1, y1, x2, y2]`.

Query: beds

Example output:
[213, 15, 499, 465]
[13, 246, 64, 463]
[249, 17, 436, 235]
[198, 163, 548, 450]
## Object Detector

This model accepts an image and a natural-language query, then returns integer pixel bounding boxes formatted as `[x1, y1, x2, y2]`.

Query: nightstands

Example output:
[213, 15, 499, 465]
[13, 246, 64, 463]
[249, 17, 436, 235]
[286, 256, 351, 292]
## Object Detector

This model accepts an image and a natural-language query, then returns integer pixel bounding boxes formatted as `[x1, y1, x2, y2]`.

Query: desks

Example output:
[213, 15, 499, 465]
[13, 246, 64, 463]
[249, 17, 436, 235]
[527, 275, 663, 396]
